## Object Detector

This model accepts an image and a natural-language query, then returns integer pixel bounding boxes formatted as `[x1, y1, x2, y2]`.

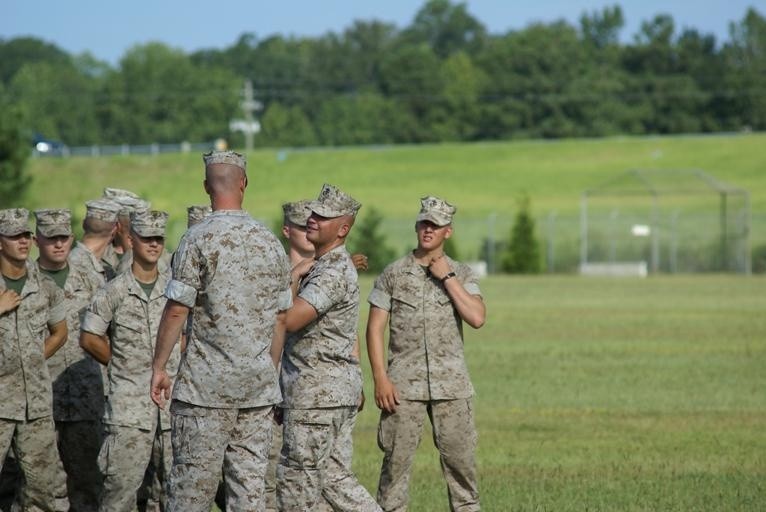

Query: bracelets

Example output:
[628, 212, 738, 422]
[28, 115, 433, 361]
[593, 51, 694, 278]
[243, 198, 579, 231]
[440, 271, 456, 284]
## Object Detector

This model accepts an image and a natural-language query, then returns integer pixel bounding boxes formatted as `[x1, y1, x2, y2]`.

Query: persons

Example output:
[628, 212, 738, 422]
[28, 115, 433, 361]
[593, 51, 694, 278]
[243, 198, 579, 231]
[150, 149, 292, 511]
[365, 195, 487, 512]
[1, 186, 389, 512]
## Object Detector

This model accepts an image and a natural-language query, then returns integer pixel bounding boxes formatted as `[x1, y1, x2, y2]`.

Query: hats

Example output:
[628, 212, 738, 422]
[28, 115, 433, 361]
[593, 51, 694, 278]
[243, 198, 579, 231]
[416, 196, 455, 227]
[203, 150, 247, 187]
[86, 188, 168, 237]
[188, 205, 213, 221]
[0, 208, 33, 237]
[282, 184, 361, 226]
[33, 208, 72, 238]
